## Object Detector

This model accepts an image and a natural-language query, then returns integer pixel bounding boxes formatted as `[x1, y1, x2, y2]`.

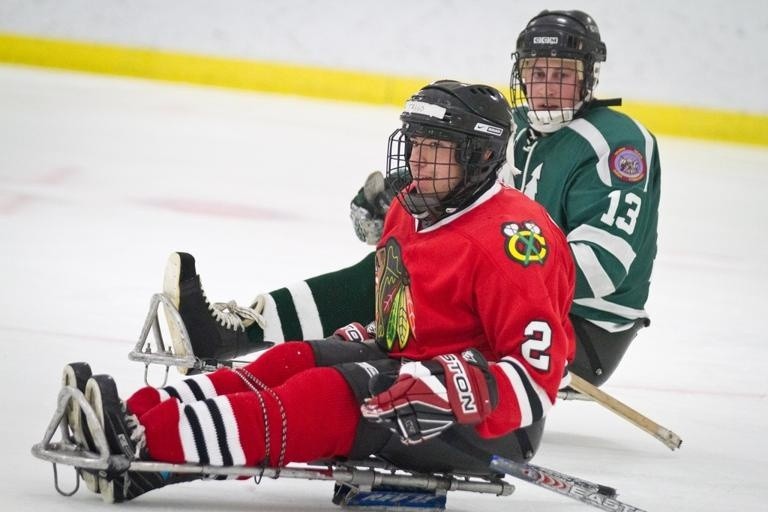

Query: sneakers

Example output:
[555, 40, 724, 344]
[64, 362, 97, 496]
[84, 374, 166, 503]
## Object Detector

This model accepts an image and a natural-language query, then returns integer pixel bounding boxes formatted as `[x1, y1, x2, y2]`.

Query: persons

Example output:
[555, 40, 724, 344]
[57, 79, 577, 507]
[131, 11, 660, 400]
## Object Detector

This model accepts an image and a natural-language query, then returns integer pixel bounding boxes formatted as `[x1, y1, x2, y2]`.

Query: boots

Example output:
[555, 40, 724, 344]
[163, 252, 265, 375]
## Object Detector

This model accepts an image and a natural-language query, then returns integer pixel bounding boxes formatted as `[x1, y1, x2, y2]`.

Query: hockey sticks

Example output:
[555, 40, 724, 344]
[369, 376, 642, 512]
[364, 170, 683, 453]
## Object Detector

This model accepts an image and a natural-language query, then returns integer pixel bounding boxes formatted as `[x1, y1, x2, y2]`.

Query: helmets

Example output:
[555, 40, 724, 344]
[517, 9, 607, 102]
[399, 79, 512, 188]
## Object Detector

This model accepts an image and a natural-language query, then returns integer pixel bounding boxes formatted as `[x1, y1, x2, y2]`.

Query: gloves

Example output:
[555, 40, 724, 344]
[360, 349, 497, 447]
[351, 170, 412, 246]
